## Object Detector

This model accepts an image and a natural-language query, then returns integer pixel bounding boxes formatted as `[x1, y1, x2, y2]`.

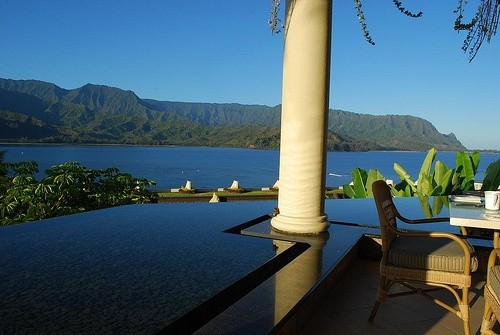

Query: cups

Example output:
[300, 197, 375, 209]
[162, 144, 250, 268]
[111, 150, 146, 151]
[484, 190, 499, 210]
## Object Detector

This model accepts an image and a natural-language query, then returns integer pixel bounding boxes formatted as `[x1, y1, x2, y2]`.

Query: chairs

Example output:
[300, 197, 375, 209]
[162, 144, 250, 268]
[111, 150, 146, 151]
[367, 180, 479, 335]
[481, 248, 500, 335]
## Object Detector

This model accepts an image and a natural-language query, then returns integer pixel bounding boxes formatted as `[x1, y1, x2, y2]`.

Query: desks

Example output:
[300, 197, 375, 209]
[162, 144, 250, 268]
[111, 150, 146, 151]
[447, 192, 500, 248]
